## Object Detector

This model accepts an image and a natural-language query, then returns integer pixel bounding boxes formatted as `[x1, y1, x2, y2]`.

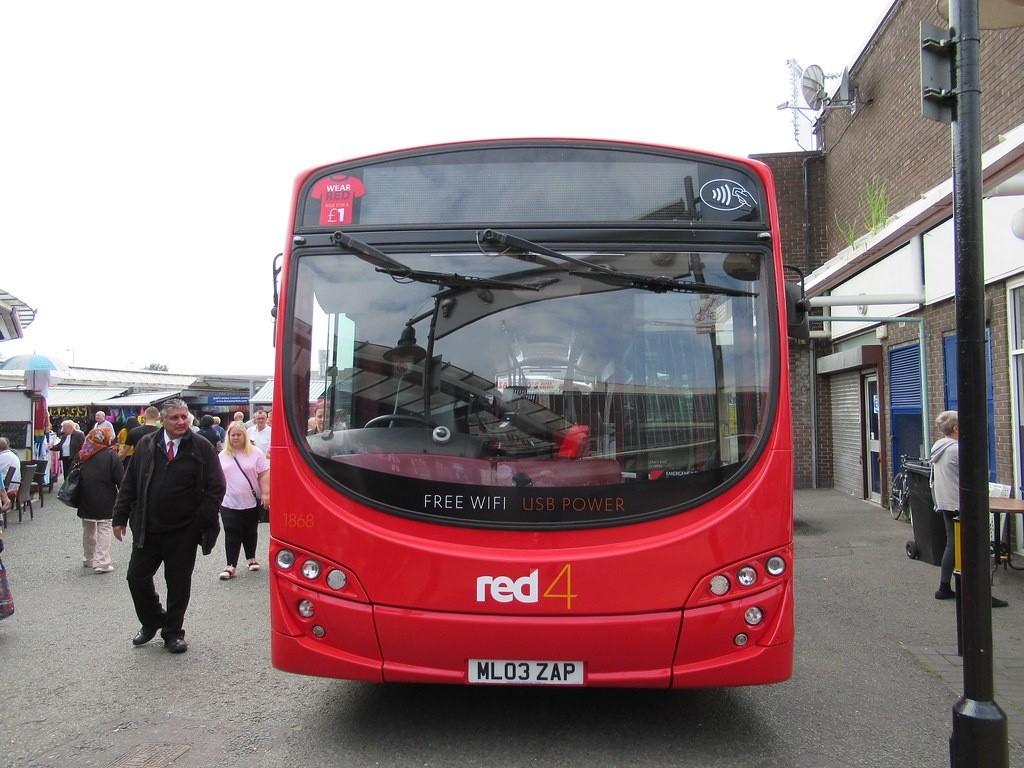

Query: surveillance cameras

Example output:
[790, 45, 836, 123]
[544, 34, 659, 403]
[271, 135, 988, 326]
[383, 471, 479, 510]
[777, 101, 790, 109]
[432, 425, 450, 445]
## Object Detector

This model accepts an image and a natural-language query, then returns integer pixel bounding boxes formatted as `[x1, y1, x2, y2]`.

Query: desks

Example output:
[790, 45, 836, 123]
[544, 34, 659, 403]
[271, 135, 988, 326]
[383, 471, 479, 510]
[990, 497, 1024, 586]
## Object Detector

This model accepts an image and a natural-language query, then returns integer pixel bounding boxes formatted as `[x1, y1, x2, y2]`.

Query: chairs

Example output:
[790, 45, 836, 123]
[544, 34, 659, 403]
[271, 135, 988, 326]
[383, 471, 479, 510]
[26, 459, 48, 508]
[0, 466, 16, 530]
[3, 464, 38, 524]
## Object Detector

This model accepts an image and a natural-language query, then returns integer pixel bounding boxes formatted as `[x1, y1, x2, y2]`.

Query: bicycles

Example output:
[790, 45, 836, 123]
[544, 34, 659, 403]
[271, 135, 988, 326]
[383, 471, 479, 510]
[888, 454, 920, 521]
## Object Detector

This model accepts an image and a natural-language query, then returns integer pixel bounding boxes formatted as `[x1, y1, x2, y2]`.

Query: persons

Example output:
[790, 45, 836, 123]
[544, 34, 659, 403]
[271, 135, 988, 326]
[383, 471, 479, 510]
[94, 411, 116, 441]
[118, 402, 351, 472]
[76, 427, 125, 573]
[0, 437, 21, 493]
[0, 462, 11, 511]
[112, 399, 227, 653]
[928, 410, 1009, 607]
[49, 420, 85, 482]
[217, 421, 271, 579]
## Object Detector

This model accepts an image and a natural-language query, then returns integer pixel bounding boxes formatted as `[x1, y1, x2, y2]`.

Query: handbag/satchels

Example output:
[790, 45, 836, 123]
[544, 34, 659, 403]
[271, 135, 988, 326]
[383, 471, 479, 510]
[56, 460, 82, 508]
[0, 559, 14, 620]
[257, 499, 269, 522]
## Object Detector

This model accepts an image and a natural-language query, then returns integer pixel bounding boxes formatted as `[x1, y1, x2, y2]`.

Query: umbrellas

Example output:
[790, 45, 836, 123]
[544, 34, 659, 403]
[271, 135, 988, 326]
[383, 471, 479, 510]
[0, 350, 57, 388]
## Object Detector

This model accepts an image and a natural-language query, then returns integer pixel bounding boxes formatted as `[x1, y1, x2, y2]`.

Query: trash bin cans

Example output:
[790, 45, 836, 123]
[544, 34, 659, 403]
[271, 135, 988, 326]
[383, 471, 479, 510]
[900, 460, 947, 566]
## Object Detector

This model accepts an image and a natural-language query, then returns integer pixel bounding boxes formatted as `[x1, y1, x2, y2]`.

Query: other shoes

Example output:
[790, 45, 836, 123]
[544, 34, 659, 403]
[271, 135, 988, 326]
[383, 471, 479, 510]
[246, 562, 259, 571]
[992, 598, 1008, 607]
[220, 566, 236, 579]
[935, 590, 956, 599]
[95, 565, 113, 574]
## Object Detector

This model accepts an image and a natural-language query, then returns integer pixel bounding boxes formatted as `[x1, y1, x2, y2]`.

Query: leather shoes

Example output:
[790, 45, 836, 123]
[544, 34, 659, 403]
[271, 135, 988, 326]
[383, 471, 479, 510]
[133, 610, 167, 645]
[164, 638, 187, 652]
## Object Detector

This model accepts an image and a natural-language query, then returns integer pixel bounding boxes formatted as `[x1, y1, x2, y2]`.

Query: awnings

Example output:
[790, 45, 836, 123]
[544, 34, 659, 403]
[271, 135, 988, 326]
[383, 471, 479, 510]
[94, 391, 181, 406]
[46, 386, 129, 407]
[247, 379, 332, 404]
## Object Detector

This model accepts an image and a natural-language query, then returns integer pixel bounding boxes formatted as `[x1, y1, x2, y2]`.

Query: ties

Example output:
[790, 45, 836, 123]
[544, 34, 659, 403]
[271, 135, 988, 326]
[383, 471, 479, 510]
[167, 441, 175, 462]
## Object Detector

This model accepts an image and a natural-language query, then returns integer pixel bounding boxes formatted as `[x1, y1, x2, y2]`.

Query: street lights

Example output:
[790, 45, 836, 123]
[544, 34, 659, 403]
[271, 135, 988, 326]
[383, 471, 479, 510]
[676, 176, 761, 471]
[384, 283, 444, 427]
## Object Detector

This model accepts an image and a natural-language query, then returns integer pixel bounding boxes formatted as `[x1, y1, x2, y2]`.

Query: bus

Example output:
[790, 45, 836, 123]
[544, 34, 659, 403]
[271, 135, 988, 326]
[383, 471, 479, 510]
[266, 140, 812, 692]
